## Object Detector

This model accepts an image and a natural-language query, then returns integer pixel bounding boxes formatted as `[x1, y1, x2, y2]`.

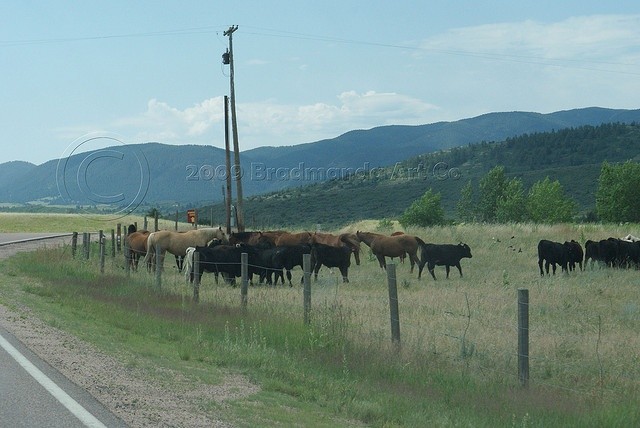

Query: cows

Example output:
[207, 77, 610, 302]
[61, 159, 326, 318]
[308, 241, 355, 284]
[258, 244, 310, 288]
[415, 237, 472, 280]
[276, 232, 311, 247]
[190, 241, 254, 290]
[538, 234, 640, 277]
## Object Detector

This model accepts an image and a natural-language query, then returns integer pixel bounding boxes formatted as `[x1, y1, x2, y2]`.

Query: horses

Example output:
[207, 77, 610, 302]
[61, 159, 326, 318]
[143, 226, 229, 277]
[307, 232, 356, 247]
[126, 232, 152, 275]
[228, 229, 287, 246]
[185, 238, 218, 286]
[356, 231, 426, 274]
